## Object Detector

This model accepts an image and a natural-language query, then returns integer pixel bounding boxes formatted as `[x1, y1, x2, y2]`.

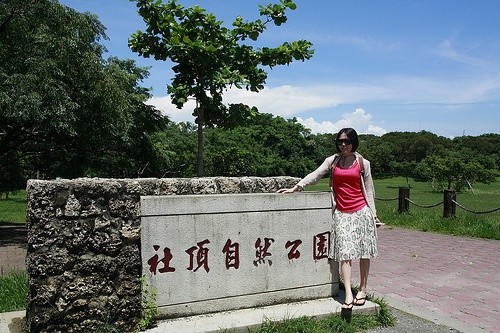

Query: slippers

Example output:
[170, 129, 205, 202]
[354, 297, 366, 305]
[342, 301, 353, 309]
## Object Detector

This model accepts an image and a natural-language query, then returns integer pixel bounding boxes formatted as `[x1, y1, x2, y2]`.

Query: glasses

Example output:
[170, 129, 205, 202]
[336, 138, 352, 145]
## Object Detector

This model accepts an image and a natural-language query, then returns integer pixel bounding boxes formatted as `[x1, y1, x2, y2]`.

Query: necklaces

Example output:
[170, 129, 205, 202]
[341, 157, 347, 167]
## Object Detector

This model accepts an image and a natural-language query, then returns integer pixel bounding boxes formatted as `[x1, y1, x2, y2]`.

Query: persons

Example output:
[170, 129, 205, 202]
[275, 128, 382, 310]
